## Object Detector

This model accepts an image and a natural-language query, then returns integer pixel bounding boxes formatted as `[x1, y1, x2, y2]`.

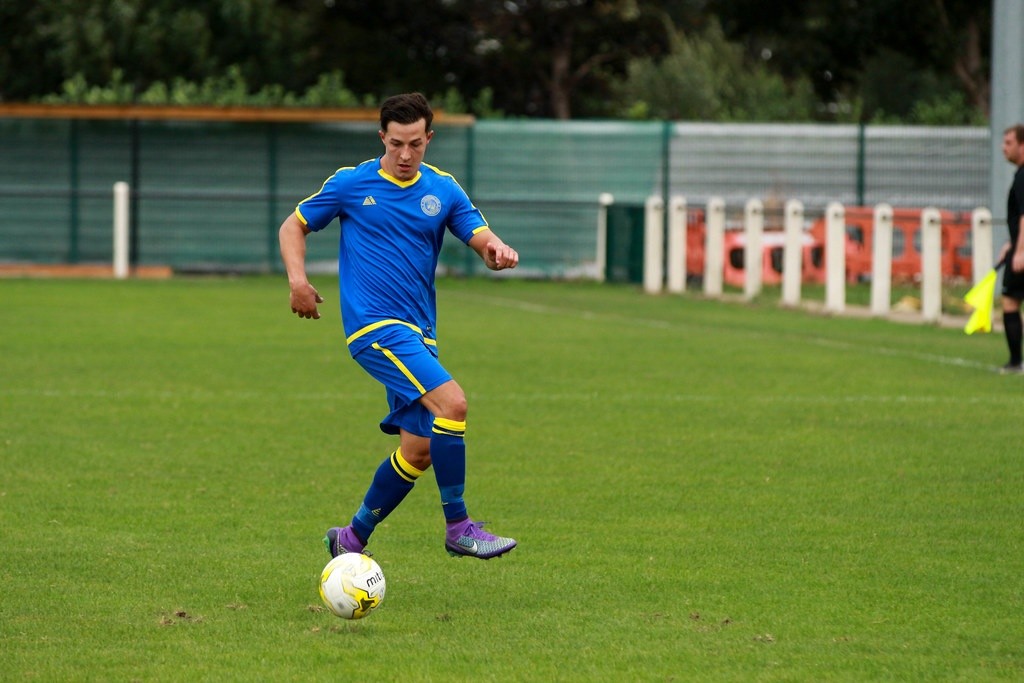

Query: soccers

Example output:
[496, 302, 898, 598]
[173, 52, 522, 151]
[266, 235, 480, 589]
[317, 553, 386, 619]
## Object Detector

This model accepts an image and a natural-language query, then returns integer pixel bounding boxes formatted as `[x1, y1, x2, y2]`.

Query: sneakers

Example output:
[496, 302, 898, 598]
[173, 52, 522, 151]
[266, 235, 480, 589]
[445, 518, 517, 560]
[323, 525, 364, 560]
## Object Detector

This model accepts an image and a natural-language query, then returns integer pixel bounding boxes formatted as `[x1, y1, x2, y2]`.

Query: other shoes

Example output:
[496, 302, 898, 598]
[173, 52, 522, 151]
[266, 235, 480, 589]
[1000, 357, 1024, 373]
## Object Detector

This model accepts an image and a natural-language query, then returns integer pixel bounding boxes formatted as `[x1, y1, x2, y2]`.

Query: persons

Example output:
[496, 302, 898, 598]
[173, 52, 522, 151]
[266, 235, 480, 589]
[993, 123, 1024, 370]
[277, 90, 521, 563]
